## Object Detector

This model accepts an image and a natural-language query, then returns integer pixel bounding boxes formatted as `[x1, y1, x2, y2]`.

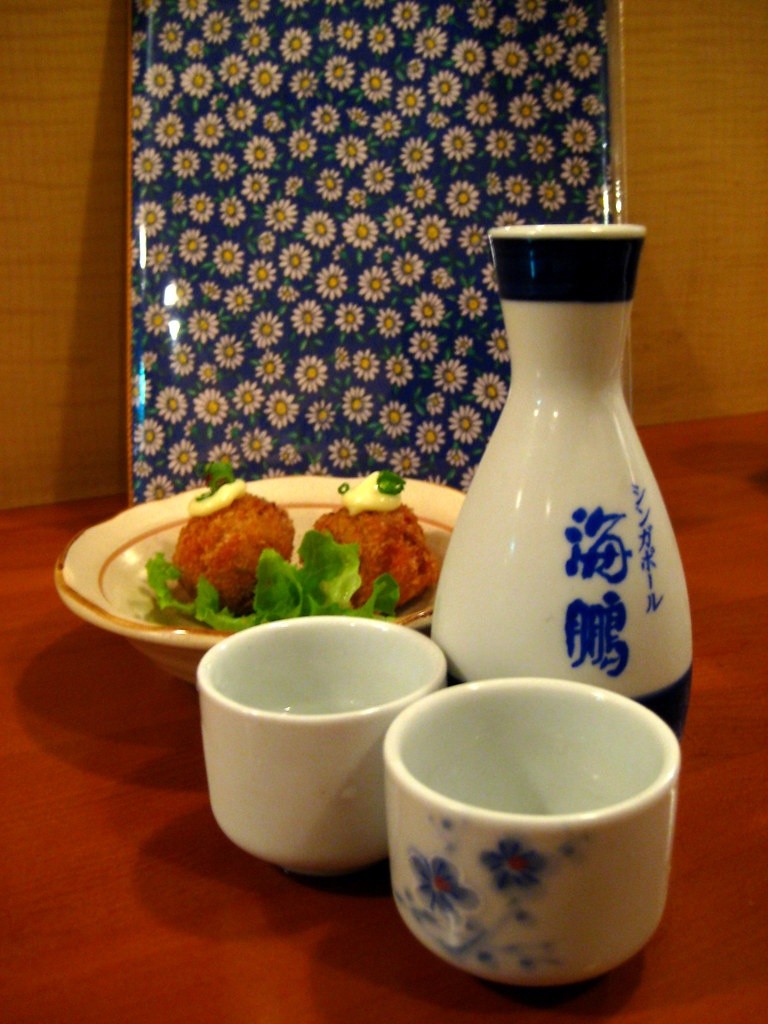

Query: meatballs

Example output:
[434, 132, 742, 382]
[310, 504, 438, 613]
[170, 492, 296, 611]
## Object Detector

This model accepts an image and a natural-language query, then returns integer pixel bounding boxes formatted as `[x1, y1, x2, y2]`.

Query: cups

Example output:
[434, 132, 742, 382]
[197, 615, 447, 876]
[387, 677, 681, 987]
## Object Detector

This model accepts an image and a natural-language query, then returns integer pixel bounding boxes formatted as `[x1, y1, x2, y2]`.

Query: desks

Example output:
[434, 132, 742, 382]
[1, 415, 767, 1024]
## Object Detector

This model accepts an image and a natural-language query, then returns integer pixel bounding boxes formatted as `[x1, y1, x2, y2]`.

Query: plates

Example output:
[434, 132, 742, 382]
[54, 475, 468, 684]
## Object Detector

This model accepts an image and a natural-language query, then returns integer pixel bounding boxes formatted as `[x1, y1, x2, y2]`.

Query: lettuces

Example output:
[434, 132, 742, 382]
[144, 533, 401, 634]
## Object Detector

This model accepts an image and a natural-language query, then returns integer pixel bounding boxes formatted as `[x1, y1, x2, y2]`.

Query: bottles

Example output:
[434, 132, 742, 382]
[432, 224, 693, 742]
